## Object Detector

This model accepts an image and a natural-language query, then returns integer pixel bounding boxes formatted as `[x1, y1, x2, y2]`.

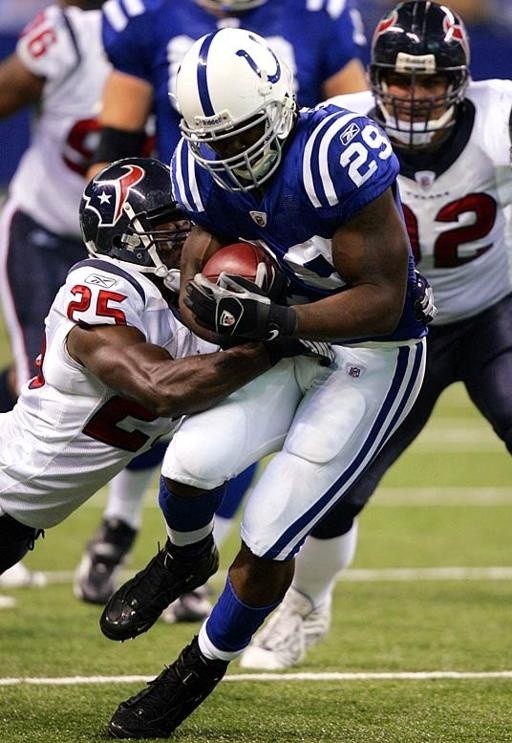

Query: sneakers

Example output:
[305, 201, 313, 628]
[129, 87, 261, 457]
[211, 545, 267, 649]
[240, 587, 332, 669]
[100, 523, 219, 643]
[163, 585, 212, 624]
[108, 632, 233, 739]
[77, 518, 138, 604]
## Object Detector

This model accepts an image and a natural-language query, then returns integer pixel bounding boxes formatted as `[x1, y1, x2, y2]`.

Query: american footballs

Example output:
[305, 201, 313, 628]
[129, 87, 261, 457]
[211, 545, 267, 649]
[204, 243, 272, 287]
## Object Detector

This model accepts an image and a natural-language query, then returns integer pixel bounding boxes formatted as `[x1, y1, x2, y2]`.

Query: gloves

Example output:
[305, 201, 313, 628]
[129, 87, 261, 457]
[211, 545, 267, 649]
[182, 270, 298, 342]
[254, 262, 288, 306]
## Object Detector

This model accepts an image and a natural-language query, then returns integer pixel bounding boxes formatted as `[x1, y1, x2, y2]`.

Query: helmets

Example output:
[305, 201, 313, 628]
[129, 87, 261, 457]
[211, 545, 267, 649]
[167, 26, 298, 191]
[365, 0, 472, 159]
[78, 155, 192, 270]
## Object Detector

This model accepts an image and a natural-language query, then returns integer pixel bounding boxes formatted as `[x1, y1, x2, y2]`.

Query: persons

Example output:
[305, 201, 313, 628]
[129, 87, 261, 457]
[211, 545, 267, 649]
[76, 1, 372, 621]
[0, 156, 285, 576]
[239, 0, 509, 669]
[101, 26, 435, 738]
[0, 0, 148, 409]
